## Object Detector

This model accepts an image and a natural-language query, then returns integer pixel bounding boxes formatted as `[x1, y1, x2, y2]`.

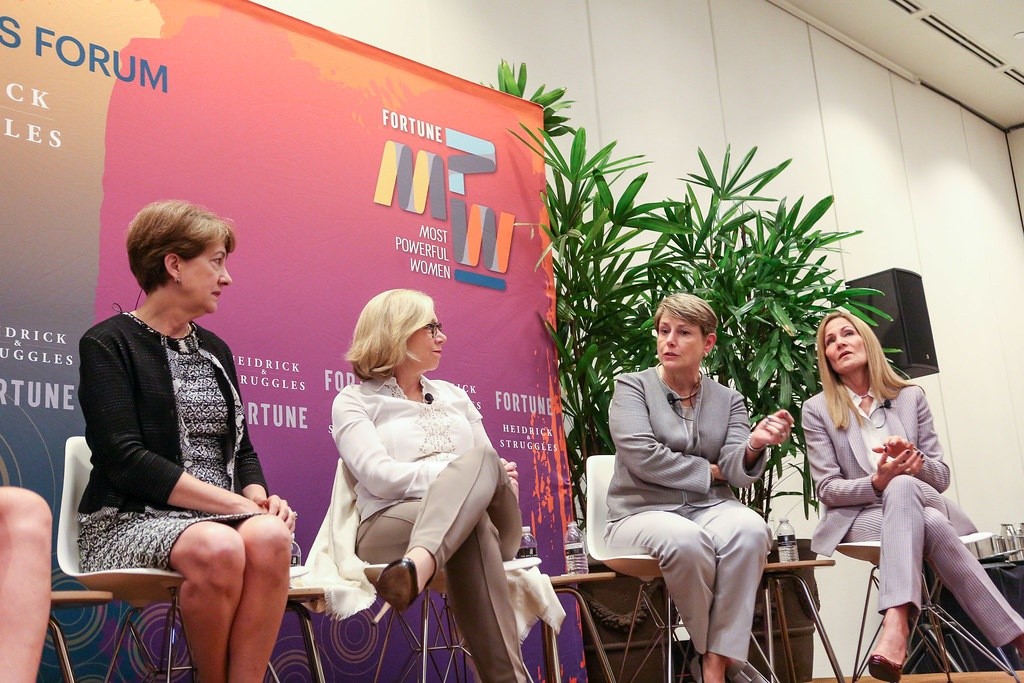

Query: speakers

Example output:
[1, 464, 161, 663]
[846, 267, 940, 381]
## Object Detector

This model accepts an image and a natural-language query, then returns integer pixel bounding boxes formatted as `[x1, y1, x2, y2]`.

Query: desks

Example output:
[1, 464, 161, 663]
[754, 559, 843, 683]
[42, 589, 113, 682]
[509, 571, 615, 683]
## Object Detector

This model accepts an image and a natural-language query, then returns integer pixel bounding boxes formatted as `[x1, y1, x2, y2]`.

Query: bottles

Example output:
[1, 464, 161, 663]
[564, 521, 590, 575]
[515, 526, 538, 559]
[288, 533, 301, 567]
[776, 517, 799, 563]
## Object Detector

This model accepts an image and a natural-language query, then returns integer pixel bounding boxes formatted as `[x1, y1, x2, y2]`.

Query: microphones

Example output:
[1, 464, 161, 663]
[883, 399, 892, 409]
[667, 393, 675, 407]
[425, 393, 434, 404]
[167, 338, 180, 352]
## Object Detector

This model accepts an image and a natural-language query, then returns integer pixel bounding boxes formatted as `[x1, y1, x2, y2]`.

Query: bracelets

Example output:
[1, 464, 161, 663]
[747, 432, 766, 452]
[711, 468, 715, 483]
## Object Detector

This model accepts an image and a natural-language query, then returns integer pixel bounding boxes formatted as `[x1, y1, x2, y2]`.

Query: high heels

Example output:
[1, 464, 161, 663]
[868, 645, 909, 683]
[371, 557, 419, 627]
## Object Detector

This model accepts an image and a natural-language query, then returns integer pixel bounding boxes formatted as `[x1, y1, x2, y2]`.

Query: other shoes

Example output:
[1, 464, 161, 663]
[690, 654, 704, 683]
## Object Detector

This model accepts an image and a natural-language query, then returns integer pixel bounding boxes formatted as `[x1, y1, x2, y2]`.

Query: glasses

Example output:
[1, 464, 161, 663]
[425, 322, 443, 338]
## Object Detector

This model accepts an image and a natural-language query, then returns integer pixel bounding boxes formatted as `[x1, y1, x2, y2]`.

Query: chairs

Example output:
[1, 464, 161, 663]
[586, 453, 780, 683]
[892, 571, 894, 574]
[335, 456, 543, 683]
[57, 436, 347, 683]
[835, 528, 1024, 683]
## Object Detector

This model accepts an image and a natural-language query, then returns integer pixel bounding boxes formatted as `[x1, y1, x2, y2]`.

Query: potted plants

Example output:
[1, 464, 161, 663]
[479, 57, 864, 683]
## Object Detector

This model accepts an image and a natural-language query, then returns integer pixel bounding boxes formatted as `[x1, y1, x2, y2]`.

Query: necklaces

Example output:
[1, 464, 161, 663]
[660, 376, 701, 401]
[858, 394, 869, 398]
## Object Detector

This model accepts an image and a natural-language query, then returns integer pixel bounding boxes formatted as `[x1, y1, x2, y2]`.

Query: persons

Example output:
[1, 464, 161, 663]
[330, 288, 535, 683]
[0, 484, 54, 683]
[801, 312, 1024, 683]
[605, 293, 794, 683]
[78, 199, 297, 683]
